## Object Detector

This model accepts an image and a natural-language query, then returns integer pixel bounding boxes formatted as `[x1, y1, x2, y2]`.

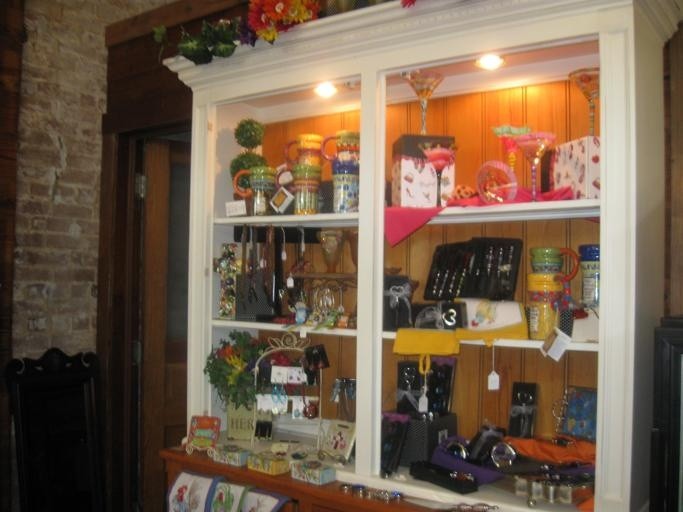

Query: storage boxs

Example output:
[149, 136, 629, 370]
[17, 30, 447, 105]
[392, 133, 456, 208]
[540, 135, 601, 200]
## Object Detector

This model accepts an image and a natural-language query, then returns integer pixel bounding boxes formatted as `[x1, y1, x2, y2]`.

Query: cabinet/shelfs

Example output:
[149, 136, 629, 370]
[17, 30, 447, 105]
[162, 0, 682, 512]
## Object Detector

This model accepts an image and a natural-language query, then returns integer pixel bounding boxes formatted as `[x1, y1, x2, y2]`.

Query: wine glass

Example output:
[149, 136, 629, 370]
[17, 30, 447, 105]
[569, 67, 601, 136]
[519, 135, 553, 203]
[421, 143, 456, 207]
[406, 72, 444, 137]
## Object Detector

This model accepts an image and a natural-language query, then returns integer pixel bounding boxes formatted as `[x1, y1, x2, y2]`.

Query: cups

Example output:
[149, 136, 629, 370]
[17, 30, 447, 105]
[286, 135, 322, 180]
[234, 166, 276, 215]
[292, 180, 317, 215]
[530, 245, 580, 287]
[332, 165, 360, 214]
[528, 274, 560, 341]
[323, 131, 358, 164]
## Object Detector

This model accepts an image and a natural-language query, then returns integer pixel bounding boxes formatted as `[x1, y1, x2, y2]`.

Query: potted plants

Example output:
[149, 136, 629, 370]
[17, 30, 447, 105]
[229, 117, 268, 216]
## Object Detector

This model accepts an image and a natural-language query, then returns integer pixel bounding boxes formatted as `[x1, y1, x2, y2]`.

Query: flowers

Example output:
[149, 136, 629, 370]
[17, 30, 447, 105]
[174, 1, 322, 67]
[203, 328, 273, 413]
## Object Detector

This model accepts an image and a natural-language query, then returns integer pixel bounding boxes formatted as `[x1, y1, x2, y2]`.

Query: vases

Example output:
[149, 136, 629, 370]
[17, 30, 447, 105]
[224, 393, 258, 440]
